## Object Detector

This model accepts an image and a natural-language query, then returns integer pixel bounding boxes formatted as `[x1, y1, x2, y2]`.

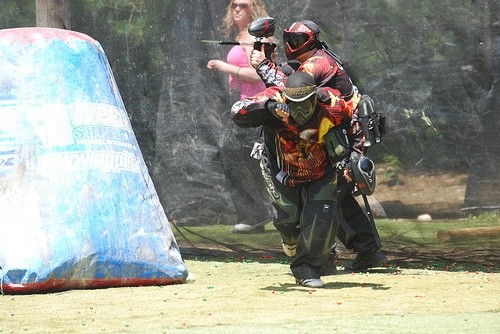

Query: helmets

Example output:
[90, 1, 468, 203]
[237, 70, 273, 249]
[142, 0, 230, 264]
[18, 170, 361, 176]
[283, 72, 316, 125]
[283, 20, 320, 59]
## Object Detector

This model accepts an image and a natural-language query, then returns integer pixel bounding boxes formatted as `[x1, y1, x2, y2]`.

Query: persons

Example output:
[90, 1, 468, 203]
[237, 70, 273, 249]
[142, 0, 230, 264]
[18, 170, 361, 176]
[250, 20, 386, 276]
[230, 72, 368, 287]
[207, 0, 279, 233]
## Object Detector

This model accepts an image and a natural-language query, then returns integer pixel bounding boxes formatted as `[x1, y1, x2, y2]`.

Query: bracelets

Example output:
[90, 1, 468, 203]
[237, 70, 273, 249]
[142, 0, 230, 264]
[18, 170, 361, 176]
[237, 67, 241, 77]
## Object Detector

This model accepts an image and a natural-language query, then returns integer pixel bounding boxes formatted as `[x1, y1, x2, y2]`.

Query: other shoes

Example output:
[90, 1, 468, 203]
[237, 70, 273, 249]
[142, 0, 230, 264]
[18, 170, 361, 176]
[344, 249, 388, 273]
[231, 218, 272, 235]
[296, 277, 324, 287]
[281, 239, 297, 257]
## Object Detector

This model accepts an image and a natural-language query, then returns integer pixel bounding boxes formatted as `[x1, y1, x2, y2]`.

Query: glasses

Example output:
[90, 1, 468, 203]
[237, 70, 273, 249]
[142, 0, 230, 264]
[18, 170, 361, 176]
[230, 3, 249, 10]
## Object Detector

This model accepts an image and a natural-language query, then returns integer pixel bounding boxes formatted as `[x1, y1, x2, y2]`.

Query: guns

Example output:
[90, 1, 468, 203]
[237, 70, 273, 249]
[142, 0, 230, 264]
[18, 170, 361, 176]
[219, 16, 277, 61]
[348, 154, 384, 248]
